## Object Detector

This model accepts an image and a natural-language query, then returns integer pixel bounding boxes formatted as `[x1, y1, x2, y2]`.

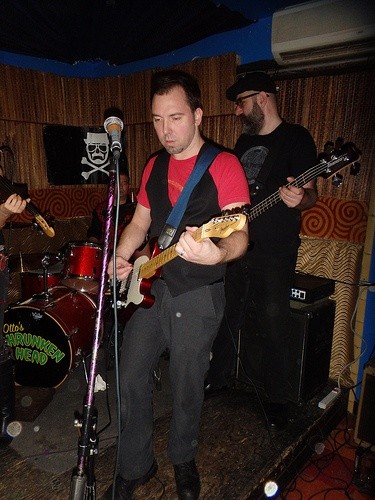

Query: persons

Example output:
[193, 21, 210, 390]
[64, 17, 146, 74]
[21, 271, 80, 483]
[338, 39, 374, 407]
[96, 71, 253, 500]
[0, 176, 33, 449]
[202, 69, 318, 432]
[88, 174, 147, 245]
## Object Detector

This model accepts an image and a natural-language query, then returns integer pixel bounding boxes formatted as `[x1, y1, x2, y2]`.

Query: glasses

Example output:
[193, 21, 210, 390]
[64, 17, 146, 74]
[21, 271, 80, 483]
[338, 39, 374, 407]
[233, 92, 270, 111]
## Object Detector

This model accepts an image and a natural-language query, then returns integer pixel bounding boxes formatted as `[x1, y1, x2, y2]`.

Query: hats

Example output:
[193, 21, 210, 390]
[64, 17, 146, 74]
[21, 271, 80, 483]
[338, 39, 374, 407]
[225, 72, 276, 102]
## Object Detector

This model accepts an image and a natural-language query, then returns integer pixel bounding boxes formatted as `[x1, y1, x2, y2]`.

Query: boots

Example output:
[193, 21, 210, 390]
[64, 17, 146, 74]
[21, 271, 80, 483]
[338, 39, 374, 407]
[0, 359, 15, 449]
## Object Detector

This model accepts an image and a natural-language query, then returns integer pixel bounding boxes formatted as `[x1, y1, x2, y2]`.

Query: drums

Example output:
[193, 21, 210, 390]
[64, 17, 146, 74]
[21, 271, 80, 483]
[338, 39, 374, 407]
[3, 285, 105, 390]
[26, 273, 59, 297]
[60, 240, 106, 293]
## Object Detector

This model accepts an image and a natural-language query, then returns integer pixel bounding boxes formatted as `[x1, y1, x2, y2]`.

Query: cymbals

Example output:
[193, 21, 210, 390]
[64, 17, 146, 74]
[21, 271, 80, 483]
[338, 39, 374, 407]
[2, 221, 34, 230]
[4, 251, 61, 273]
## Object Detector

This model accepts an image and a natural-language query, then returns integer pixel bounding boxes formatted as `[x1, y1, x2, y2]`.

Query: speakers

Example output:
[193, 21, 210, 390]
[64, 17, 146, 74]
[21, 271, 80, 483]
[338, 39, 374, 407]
[199, 282, 336, 406]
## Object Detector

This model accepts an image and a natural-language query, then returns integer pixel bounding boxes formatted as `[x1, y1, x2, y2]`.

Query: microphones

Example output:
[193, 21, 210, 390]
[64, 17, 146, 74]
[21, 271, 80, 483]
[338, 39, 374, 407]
[103, 116, 124, 158]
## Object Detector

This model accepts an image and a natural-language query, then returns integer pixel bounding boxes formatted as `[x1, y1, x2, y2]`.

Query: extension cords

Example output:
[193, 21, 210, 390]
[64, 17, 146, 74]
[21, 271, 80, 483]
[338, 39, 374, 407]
[318, 387, 342, 409]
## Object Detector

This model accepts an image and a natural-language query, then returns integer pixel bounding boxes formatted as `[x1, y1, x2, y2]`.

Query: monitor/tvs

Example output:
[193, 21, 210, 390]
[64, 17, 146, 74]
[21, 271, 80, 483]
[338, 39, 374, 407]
[42, 124, 121, 186]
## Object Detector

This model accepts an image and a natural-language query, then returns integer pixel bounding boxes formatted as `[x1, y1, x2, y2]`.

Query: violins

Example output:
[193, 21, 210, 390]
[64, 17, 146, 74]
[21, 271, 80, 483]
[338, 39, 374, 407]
[0, 175, 57, 239]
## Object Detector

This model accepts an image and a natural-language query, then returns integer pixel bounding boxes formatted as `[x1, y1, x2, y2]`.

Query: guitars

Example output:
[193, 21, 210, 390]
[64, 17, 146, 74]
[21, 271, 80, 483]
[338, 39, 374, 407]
[109, 202, 252, 322]
[248, 136, 363, 223]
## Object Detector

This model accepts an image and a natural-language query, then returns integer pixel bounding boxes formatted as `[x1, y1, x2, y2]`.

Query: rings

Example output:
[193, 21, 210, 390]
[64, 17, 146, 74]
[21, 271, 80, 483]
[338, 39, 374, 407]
[180, 249, 185, 257]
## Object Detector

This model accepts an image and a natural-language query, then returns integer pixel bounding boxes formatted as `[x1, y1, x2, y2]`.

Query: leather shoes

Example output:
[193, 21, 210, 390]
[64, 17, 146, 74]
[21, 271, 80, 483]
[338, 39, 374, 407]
[100, 457, 158, 500]
[173, 458, 201, 500]
[204, 379, 236, 398]
[266, 401, 287, 431]
